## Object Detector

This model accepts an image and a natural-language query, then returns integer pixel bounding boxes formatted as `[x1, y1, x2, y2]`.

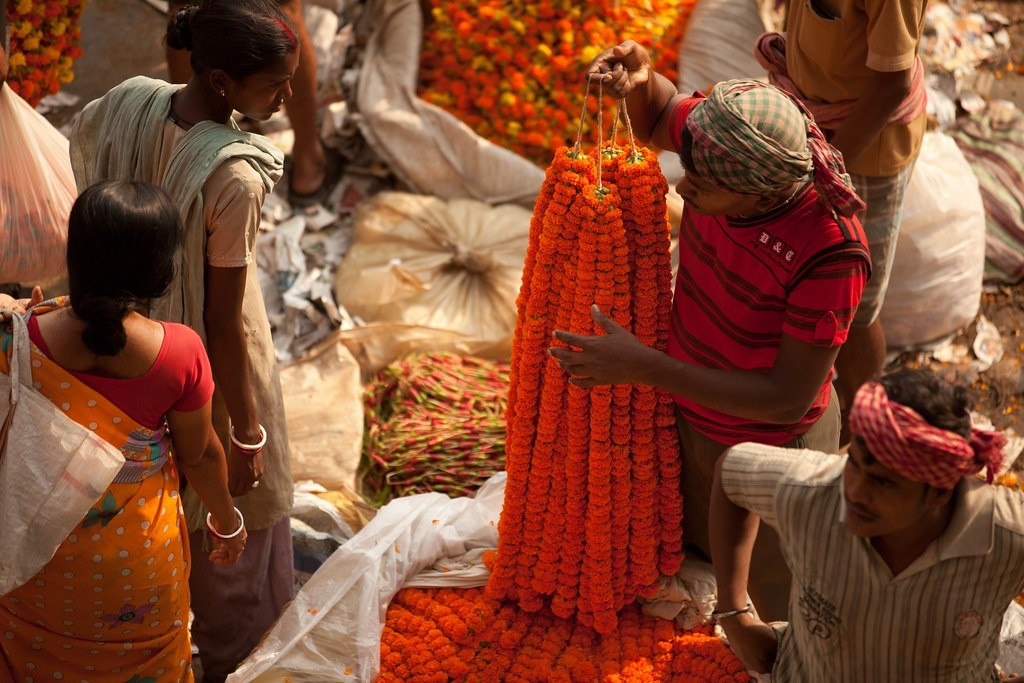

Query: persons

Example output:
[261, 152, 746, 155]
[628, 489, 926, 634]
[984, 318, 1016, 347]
[753, 0, 930, 403]
[548, 35, 872, 621]
[67, 1, 299, 683]
[164, 0, 344, 208]
[707, 365, 1023, 683]
[0, 179, 247, 683]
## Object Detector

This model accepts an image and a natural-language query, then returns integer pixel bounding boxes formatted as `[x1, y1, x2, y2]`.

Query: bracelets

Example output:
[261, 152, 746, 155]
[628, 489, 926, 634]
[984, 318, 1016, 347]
[711, 604, 752, 626]
[230, 422, 267, 455]
[206, 506, 244, 539]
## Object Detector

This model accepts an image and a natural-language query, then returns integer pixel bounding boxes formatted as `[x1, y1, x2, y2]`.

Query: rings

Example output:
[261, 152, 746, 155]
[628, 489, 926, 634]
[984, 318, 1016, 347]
[252, 481, 260, 488]
